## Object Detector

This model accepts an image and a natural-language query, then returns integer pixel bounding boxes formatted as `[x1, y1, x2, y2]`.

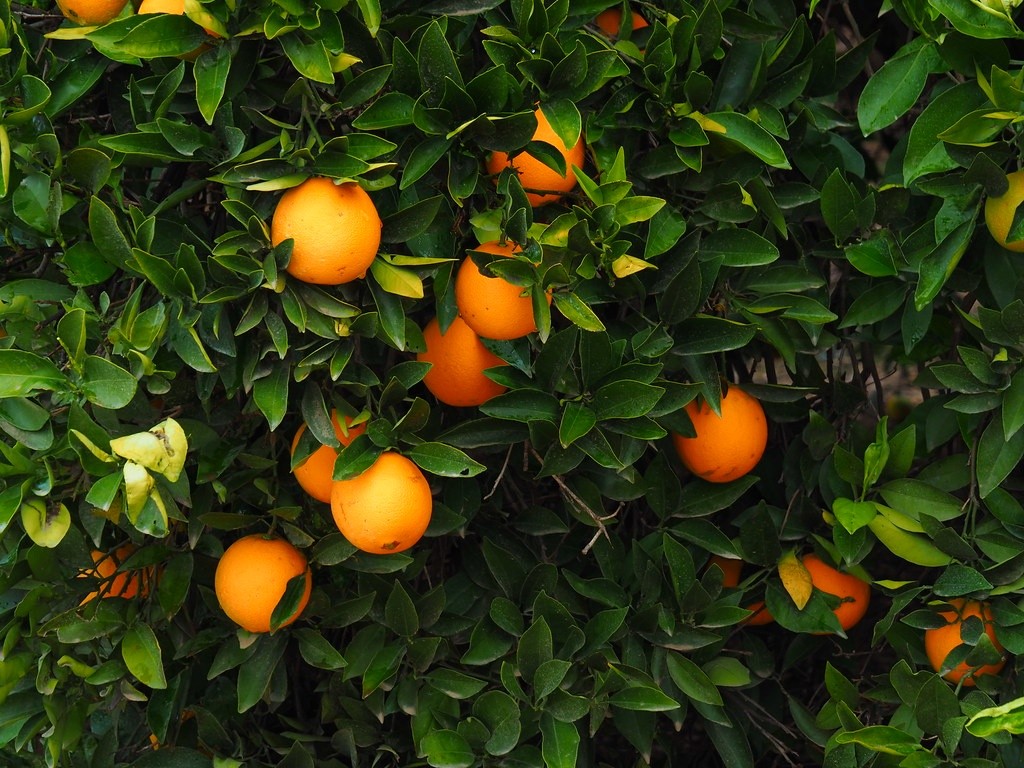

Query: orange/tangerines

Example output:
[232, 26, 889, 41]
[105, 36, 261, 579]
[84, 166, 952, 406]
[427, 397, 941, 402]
[51, 0, 1024, 689]
